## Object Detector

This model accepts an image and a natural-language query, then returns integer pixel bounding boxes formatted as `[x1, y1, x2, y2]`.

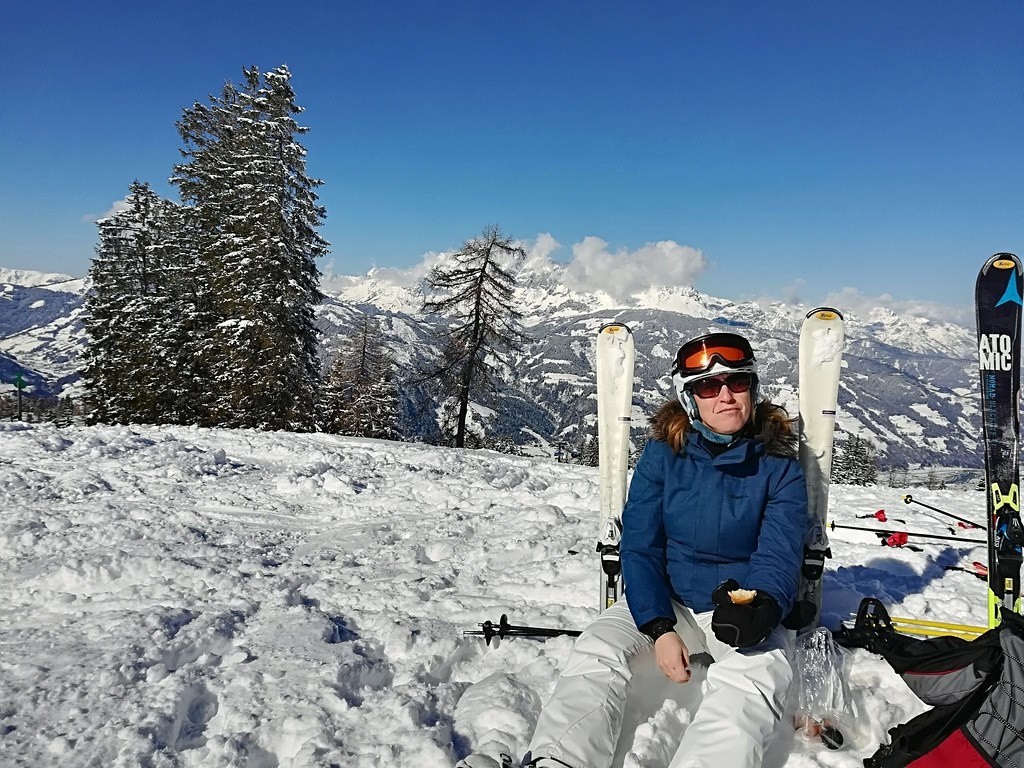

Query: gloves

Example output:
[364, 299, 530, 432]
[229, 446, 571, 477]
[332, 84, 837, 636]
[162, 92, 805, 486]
[712, 578, 782, 648]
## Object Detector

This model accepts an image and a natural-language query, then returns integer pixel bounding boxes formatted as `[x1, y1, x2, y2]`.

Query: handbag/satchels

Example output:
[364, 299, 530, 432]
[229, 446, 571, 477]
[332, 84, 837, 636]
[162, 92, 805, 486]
[829, 597, 1024, 768]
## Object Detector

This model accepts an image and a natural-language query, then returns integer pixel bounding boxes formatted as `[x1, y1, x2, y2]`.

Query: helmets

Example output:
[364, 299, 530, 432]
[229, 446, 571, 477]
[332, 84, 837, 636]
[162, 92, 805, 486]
[672, 333, 760, 420]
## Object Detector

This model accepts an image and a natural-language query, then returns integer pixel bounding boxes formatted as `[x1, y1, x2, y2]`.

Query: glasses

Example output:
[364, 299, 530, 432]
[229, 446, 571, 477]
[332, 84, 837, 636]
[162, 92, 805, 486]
[686, 372, 753, 399]
[677, 333, 756, 377]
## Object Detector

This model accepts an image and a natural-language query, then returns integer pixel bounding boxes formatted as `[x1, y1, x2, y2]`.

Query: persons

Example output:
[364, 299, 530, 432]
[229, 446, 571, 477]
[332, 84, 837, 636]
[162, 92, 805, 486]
[521, 332, 807, 768]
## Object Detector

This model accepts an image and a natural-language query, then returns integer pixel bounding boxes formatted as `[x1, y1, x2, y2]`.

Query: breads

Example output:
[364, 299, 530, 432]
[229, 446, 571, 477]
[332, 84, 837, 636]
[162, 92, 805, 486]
[727, 588, 757, 604]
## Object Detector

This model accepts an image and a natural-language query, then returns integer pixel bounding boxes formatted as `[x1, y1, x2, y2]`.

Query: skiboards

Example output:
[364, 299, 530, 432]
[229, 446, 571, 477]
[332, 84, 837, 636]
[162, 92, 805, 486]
[972, 251, 1024, 632]
[594, 305, 849, 637]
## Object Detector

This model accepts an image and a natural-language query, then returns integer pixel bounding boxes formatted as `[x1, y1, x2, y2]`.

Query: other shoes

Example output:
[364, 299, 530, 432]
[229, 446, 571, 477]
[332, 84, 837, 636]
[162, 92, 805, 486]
[456, 753, 550, 768]
